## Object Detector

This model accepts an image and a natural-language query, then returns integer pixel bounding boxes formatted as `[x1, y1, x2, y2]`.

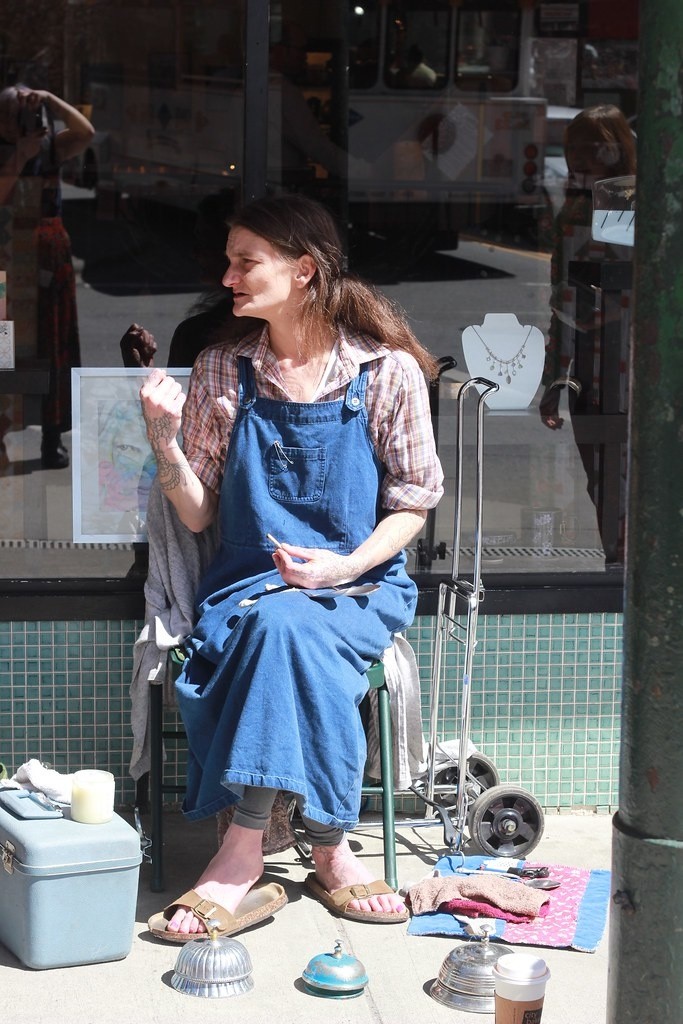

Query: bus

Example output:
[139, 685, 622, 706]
[58, 0, 548, 241]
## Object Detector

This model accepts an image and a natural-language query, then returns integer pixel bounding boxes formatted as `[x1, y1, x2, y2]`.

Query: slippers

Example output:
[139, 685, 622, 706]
[148, 881, 289, 943]
[304, 871, 410, 924]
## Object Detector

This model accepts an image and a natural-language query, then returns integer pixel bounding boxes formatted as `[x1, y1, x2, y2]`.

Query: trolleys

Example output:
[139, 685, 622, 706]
[417, 356, 457, 574]
[347, 377, 543, 858]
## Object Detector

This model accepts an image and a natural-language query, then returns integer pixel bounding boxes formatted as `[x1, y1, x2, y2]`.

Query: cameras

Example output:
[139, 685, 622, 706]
[17, 104, 42, 136]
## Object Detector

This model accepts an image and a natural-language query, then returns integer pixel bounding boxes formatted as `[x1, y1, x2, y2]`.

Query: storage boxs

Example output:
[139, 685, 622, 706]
[0, 782, 142, 970]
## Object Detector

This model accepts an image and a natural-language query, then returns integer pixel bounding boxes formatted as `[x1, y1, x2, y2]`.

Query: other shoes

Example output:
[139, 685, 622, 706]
[0, 443, 10, 470]
[40, 438, 69, 469]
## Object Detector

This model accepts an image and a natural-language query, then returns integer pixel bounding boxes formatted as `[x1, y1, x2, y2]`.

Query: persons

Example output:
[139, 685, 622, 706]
[539, 103, 634, 571]
[119, 295, 266, 369]
[0, 84, 96, 472]
[140, 192, 445, 944]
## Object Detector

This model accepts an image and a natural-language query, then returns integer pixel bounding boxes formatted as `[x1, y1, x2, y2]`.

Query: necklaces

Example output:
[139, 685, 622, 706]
[472, 324, 534, 384]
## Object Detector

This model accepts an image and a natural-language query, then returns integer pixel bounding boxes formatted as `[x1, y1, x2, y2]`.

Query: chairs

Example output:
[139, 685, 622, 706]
[130, 473, 421, 891]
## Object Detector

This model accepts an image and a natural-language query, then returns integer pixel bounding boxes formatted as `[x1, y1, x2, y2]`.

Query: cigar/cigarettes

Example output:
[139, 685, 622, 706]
[266, 533, 282, 548]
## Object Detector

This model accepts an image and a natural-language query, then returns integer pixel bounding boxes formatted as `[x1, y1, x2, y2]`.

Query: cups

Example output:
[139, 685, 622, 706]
[71, 770, 115, 824]
[492, 954, 552, 1024]
